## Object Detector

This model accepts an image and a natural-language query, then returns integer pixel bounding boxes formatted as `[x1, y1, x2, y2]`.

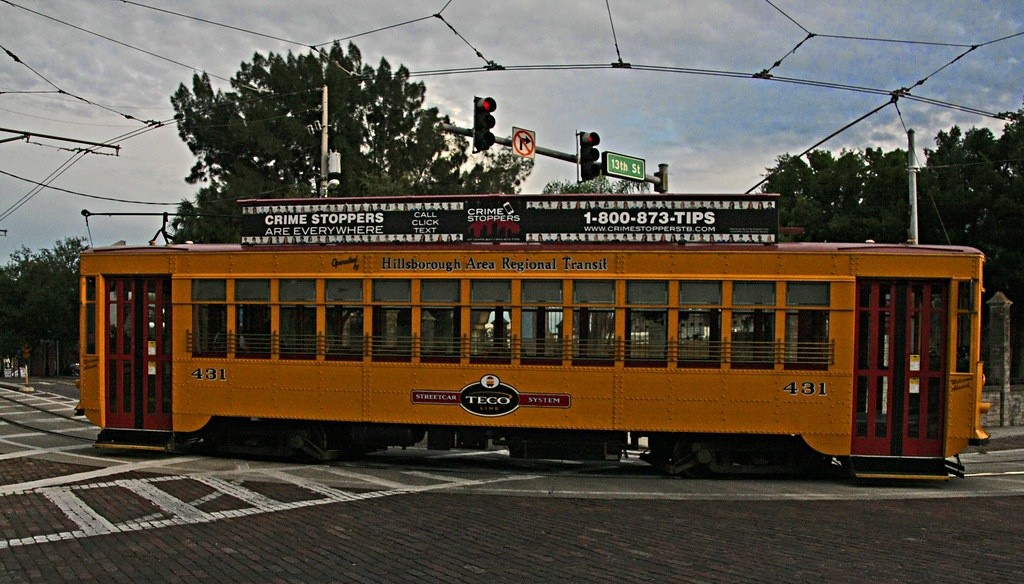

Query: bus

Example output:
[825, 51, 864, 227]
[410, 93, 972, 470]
[70, 193, 993, 484]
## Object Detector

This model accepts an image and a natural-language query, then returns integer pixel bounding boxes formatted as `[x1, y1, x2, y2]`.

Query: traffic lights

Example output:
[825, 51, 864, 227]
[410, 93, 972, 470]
[472, 95, 496, 153]
[580, 131, 601, 181]
